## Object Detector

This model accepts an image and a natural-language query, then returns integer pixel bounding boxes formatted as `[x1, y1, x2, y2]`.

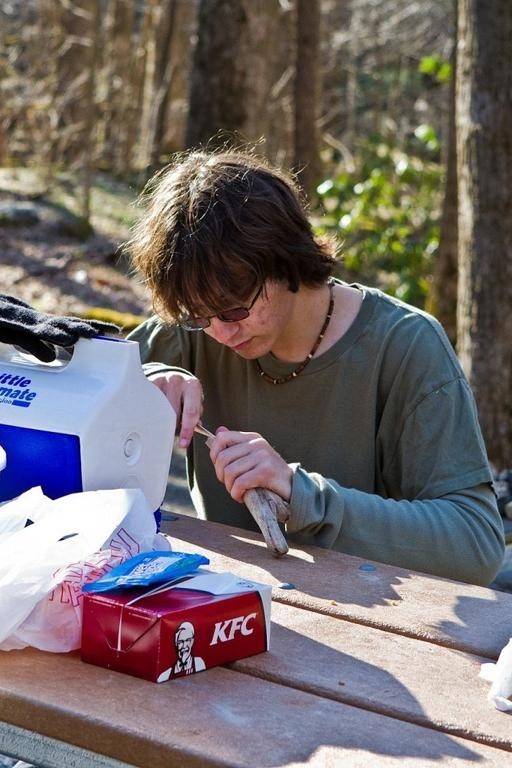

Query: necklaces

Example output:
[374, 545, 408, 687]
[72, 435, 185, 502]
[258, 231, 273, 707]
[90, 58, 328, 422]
[251, 280, 336, 383]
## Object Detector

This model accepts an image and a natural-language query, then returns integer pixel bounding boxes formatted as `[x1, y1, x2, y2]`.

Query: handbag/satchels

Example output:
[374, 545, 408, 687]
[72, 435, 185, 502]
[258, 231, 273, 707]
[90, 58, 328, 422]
[0, 486, 171, 653]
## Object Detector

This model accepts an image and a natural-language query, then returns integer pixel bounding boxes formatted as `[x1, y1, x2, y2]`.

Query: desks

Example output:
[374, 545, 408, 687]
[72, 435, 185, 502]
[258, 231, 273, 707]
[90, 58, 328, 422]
[0, 509, 512, 767]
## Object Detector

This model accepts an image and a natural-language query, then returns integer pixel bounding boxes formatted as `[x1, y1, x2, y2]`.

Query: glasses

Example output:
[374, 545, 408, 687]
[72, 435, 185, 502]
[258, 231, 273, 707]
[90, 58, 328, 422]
[175, 274, 270, 332]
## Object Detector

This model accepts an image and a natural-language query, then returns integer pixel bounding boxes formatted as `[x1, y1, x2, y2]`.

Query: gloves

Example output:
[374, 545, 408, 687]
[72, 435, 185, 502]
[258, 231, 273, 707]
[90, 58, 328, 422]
[0, 294, 119, 362]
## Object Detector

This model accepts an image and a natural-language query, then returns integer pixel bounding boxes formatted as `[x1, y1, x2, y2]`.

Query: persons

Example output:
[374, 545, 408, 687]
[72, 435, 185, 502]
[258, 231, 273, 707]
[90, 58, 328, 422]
[156, 622, 207, 683]
[116, 133, 506, 588]
